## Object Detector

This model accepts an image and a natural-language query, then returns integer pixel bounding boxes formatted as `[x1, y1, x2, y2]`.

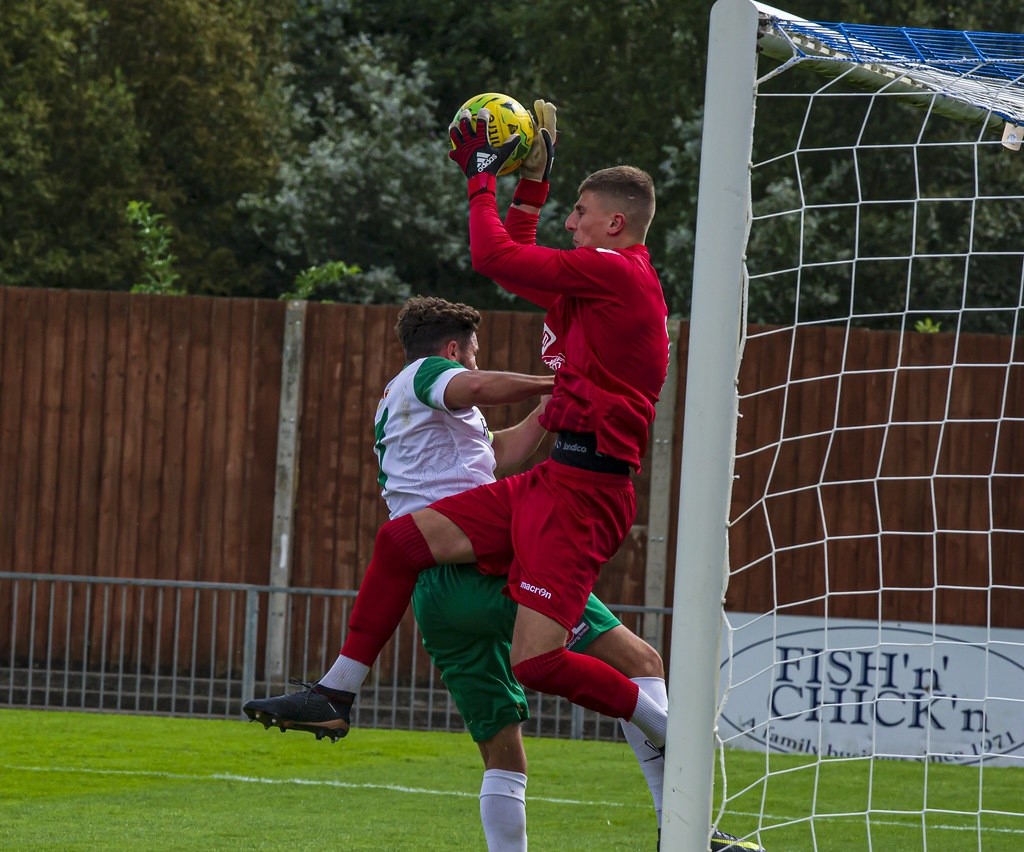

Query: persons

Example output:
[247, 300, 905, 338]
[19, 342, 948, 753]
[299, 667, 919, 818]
[372, 294, 765, 852]
[242, 95, 667, 757]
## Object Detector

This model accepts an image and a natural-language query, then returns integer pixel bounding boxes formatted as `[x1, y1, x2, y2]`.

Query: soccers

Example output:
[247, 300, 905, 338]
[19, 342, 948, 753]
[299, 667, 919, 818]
[451, 92, 534, 176]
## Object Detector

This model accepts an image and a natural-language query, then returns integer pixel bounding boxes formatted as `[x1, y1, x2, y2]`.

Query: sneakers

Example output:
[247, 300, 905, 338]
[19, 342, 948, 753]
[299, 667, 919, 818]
[657, 824, 765, 851]
[242, 677, 351, 744]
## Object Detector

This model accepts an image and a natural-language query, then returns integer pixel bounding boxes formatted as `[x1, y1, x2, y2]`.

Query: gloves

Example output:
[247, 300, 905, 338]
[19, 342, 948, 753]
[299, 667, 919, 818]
[448, 107, 520, 174]
[518, 97, 556, 181]
